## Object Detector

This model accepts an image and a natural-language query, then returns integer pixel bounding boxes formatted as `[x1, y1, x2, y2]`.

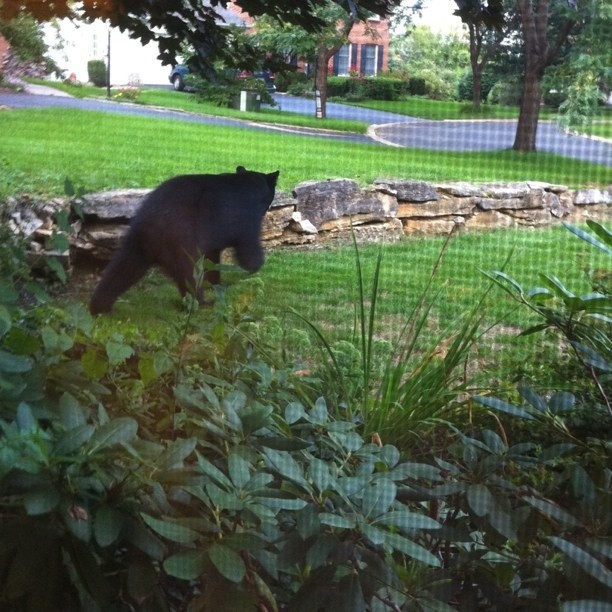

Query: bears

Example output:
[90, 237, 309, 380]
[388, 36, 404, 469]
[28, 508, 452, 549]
[88, 163, 282, 319]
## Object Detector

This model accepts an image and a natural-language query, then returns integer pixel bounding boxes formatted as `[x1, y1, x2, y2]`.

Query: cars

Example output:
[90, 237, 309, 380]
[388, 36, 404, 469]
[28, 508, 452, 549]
[169, 56, 278, 95]
